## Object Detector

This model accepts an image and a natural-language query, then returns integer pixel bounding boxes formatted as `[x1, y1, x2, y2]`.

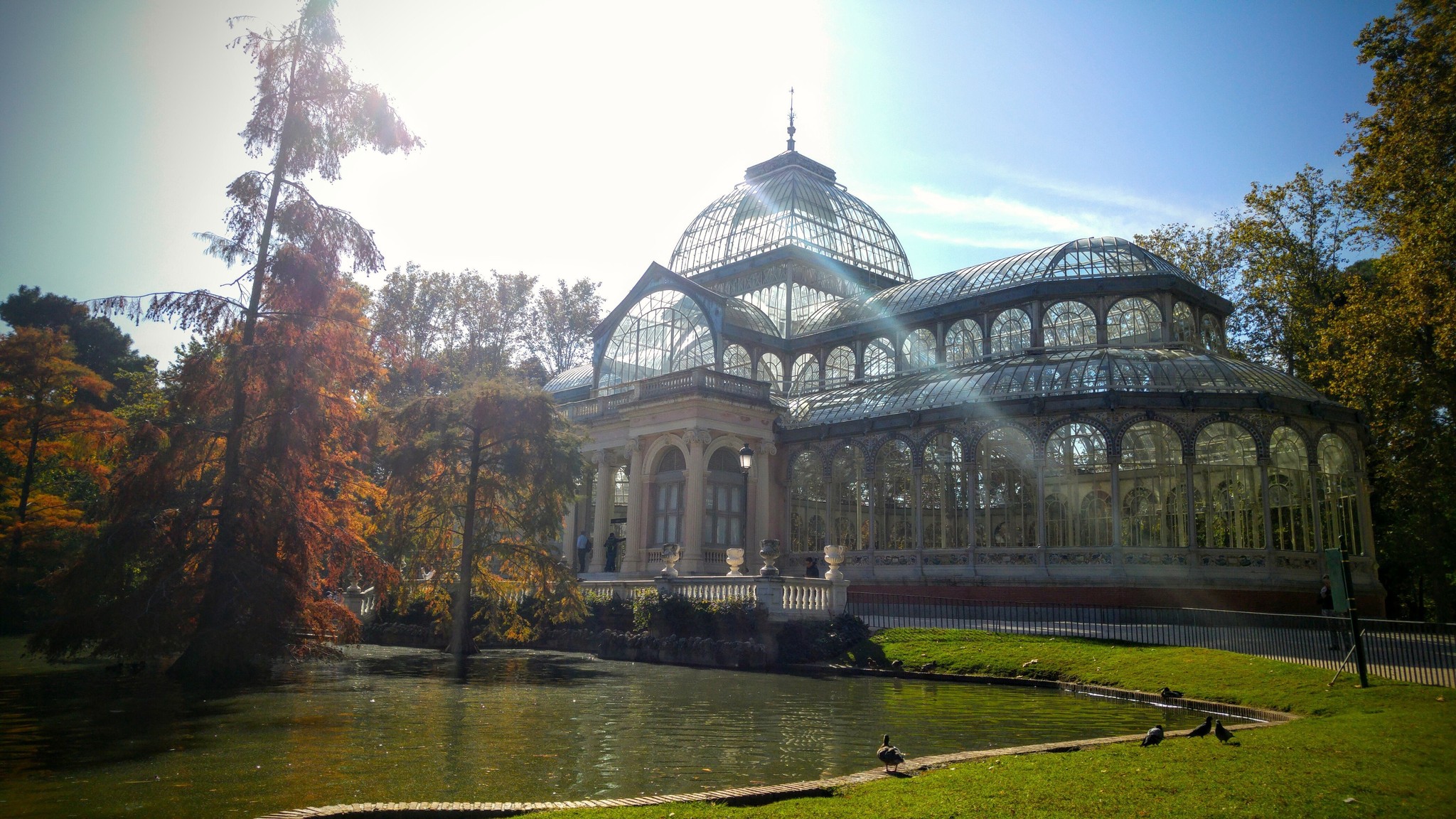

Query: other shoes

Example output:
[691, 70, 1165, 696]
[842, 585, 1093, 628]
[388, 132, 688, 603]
[612, 569, 616, 572]
[578, 571, 584, 573]
[604, 570, 609, 572]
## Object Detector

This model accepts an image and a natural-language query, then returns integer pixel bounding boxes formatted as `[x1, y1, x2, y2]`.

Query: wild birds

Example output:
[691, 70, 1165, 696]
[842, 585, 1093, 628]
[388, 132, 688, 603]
[891, 659, 904, 670]
[867, 656, 883, 670]
[1215, 720, 1238, 743]
[1161, 687, 1187, 698]
[878, 735, 906, 774]
[921, 659, 938, 674]
[1139, 724, 1166, 748]
[1185, 714, 1214, 739]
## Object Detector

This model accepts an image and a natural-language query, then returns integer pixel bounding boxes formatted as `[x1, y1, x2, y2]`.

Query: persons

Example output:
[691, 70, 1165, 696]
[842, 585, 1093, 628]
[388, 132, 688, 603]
[577, 531, 589, 574]
[602, 533, 626, 573]
[801, 557, 819, 605]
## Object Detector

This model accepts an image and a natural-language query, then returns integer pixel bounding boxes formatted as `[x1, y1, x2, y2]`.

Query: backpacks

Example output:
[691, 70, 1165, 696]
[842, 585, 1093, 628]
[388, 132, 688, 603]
[585, 537, 592, 553]
[606, 538, 614, 552]
